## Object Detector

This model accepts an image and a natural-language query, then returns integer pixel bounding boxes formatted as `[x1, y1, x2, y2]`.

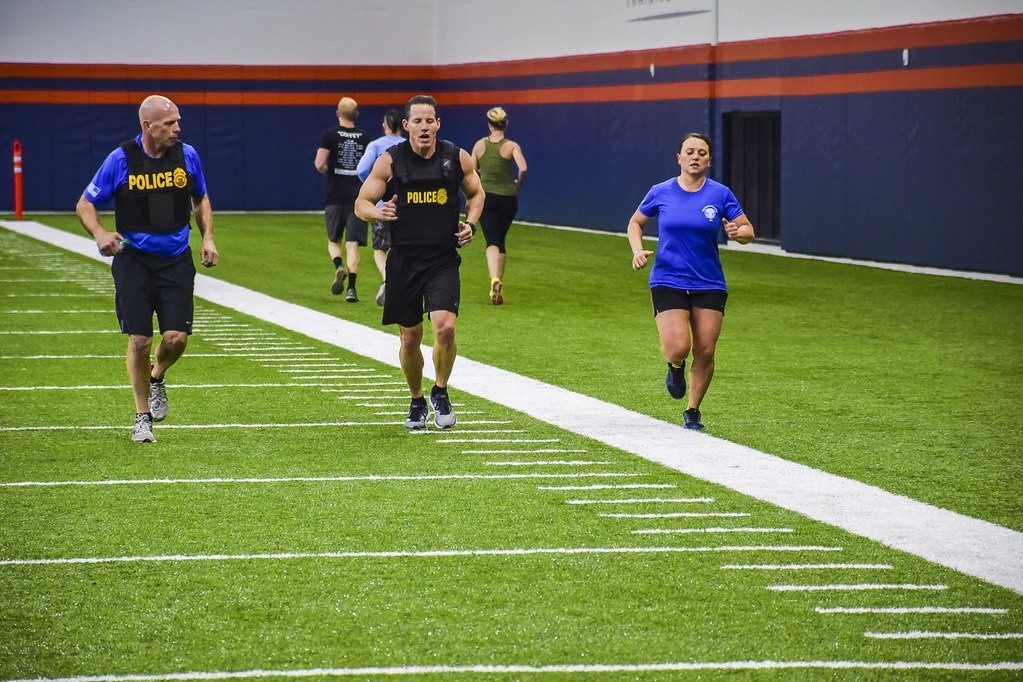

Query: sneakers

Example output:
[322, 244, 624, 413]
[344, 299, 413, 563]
[405, 400, 431, 429]
[377, 283, 385, 307]
[131, 413, 157, 444]
[331, 266, 348, 296]
[682, 408, 705, 429]
[151, 371, 169, 422]
[666, 360, 686, 400]
[346, 289, 359, 302]
[489, 277, 504, 305]
[428, 390, 456, 429]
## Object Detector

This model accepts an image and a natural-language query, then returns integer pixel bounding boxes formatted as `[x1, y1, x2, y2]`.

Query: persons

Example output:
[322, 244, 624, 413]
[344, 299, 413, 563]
[471, 107, 527, 304]
[313, 97, 368, 302]
[357, 109, 410, 308]
[353, 94, 485, 432]
[74, 96, 219, 443]
[627, 133, 755, 431]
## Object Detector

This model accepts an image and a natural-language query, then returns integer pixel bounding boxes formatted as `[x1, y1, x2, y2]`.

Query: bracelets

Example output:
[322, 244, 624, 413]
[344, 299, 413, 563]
[463, 220, 477, 235]
